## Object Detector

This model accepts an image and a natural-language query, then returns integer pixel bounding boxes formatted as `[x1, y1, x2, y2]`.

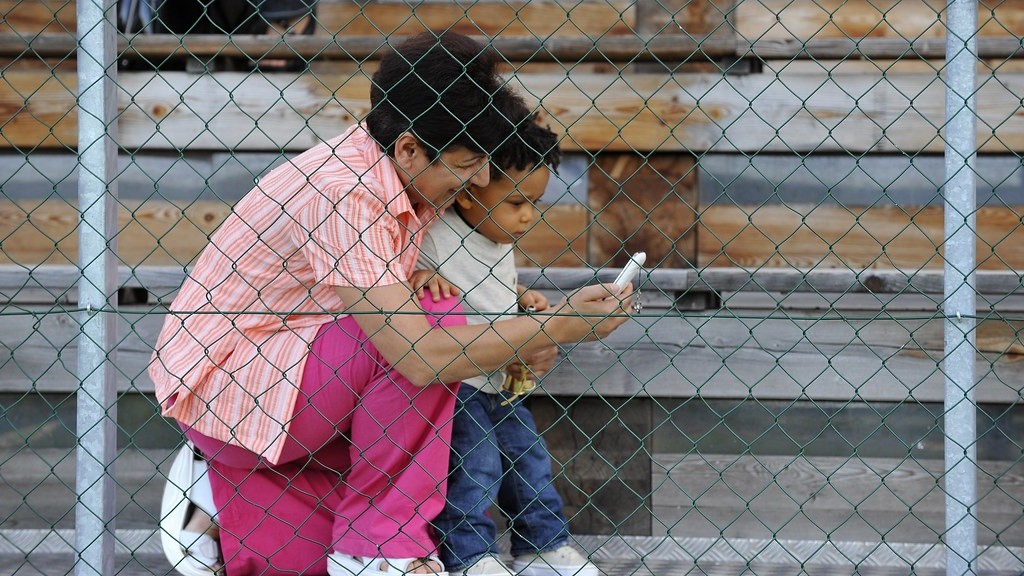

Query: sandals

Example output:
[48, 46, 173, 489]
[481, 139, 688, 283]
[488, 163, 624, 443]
[160, 440, 225, 576]
[327, 547, 450, 576]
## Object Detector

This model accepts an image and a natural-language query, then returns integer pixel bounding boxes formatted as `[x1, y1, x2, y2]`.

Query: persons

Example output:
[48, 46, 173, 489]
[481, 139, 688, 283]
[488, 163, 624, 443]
[408, 117, 609, 576]
[150, 32, 639, 576]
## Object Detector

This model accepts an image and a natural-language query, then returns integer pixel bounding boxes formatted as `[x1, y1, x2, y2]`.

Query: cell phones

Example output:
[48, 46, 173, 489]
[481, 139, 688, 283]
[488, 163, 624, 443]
[602, 250, 647, 302]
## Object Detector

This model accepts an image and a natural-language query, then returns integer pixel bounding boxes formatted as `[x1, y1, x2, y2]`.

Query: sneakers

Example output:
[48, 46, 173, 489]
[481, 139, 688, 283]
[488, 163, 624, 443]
[450, 553, 518, 576]
[513, 546, 598, 576]
